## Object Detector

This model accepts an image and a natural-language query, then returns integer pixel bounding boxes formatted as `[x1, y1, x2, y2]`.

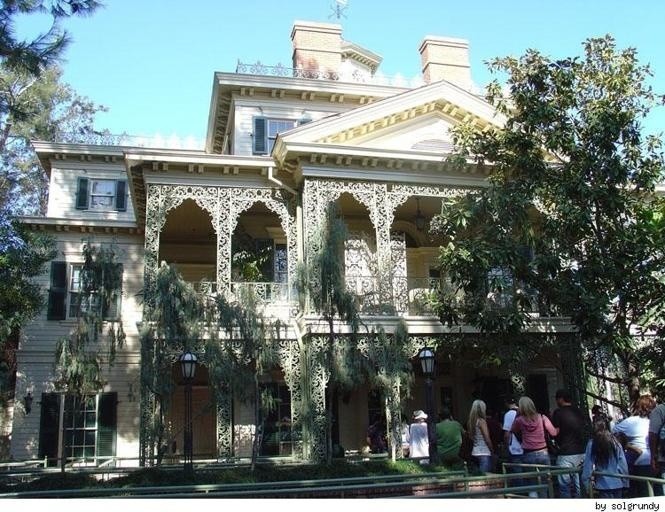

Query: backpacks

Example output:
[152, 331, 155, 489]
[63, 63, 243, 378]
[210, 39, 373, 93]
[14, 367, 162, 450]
[507, 408, 522, 444]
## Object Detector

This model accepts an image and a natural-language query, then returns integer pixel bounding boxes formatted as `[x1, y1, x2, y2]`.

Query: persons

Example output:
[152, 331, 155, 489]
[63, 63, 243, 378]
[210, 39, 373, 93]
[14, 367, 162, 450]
[366, 403, 665, 498]
[511, 396, 561, 498]
[467, 399, 493, 472]
[500, 390, 528, 493]
[550, 389, 586, 498]
[612, 394, 664, 498]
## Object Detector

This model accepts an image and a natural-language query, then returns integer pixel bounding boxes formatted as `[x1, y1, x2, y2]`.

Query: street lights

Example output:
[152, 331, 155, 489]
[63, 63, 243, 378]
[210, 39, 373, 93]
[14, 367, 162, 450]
[177, 351, 201, 485]
[414, 342, 442, 473]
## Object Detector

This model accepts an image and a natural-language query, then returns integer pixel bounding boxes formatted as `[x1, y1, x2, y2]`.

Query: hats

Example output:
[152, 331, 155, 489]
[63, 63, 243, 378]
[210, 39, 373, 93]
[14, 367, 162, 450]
[413, 410, 428, 420]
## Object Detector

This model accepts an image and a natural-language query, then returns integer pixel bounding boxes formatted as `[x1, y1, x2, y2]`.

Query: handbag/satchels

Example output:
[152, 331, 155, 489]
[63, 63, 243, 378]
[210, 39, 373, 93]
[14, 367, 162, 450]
[545, 429, 561, 456]
[458, 432, 478, 462]
